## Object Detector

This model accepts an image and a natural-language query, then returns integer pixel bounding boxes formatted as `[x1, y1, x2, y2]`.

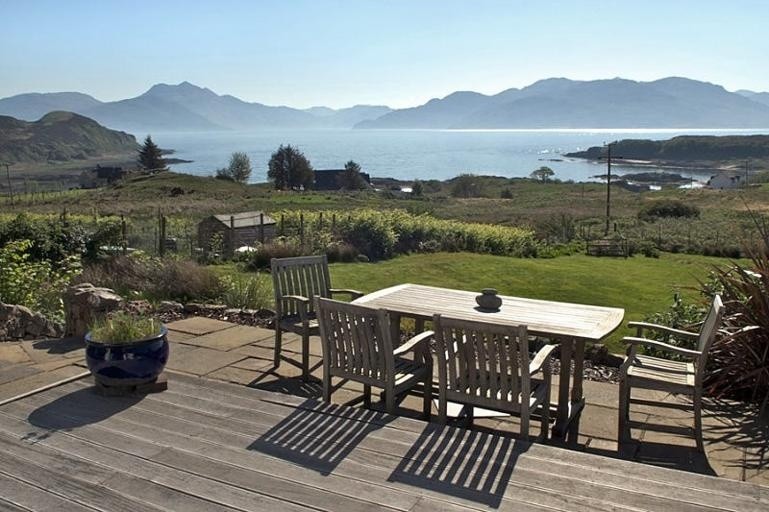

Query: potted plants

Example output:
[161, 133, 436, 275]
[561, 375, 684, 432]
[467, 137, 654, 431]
[85, 306, 168, 388]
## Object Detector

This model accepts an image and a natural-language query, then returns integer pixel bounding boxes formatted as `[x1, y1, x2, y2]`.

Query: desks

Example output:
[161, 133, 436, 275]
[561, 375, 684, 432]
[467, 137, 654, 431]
[351, 282, 627, 440]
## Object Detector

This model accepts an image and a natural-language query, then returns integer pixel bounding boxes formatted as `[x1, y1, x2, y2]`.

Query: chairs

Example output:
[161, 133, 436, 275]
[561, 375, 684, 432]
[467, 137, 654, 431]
[271, 257, 364, 382]
[434, 312, 557, 441]
[618, 293, 727, 446]
[314, 294, 434, 421]
